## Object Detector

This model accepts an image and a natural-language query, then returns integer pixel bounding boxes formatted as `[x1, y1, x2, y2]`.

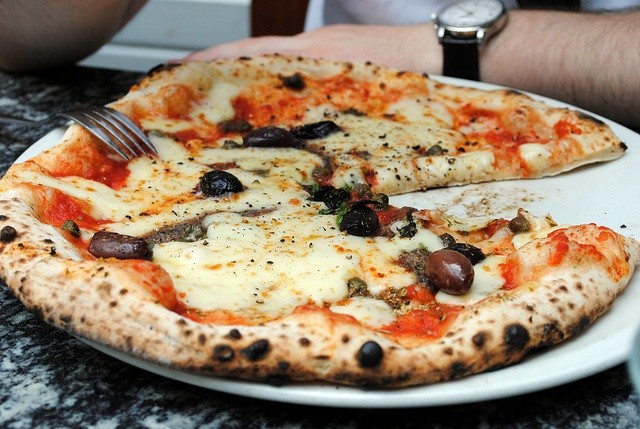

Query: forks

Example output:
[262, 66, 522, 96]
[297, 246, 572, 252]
[0, 102, 159, 162]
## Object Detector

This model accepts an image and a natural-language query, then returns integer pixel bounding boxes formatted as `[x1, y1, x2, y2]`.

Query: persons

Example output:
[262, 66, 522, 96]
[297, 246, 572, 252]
[1, 0, 638, 135]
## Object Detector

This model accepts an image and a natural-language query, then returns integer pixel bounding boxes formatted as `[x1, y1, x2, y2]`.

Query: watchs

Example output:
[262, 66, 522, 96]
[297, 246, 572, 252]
[430, 0, 510, 82]
